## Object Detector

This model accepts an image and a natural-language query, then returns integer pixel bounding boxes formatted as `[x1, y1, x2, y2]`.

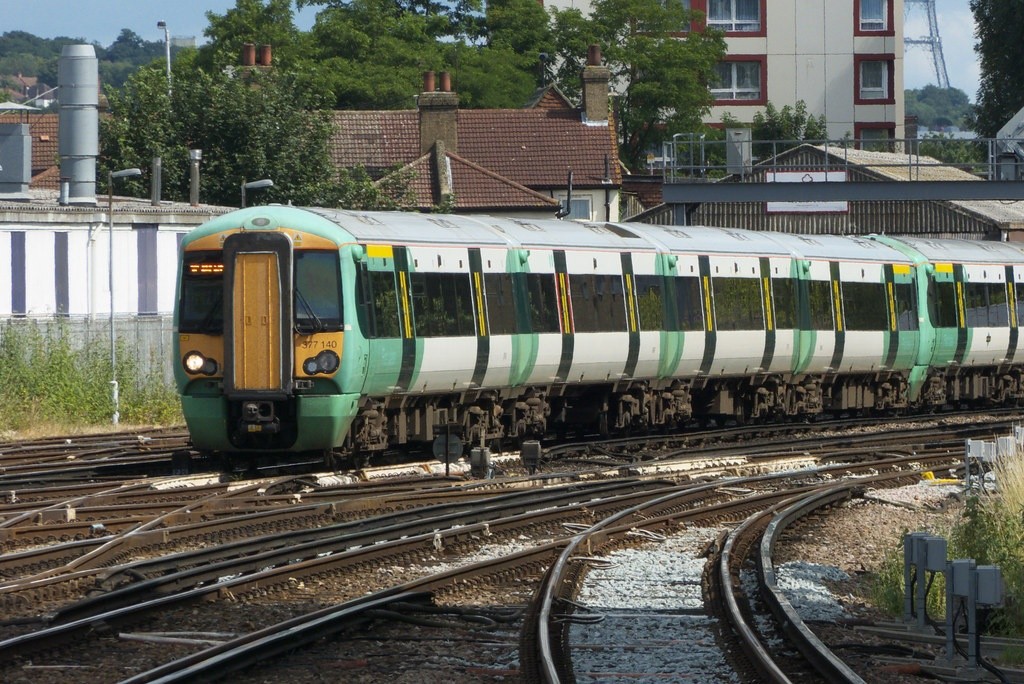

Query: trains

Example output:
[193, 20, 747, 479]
[172, 207, 1024, 468]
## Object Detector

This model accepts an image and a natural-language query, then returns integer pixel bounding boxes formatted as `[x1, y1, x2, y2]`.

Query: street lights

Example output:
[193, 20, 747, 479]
[241, 176, 273, 209]
[108, 168, 141, 426]
[157, 20, 172, 98]
